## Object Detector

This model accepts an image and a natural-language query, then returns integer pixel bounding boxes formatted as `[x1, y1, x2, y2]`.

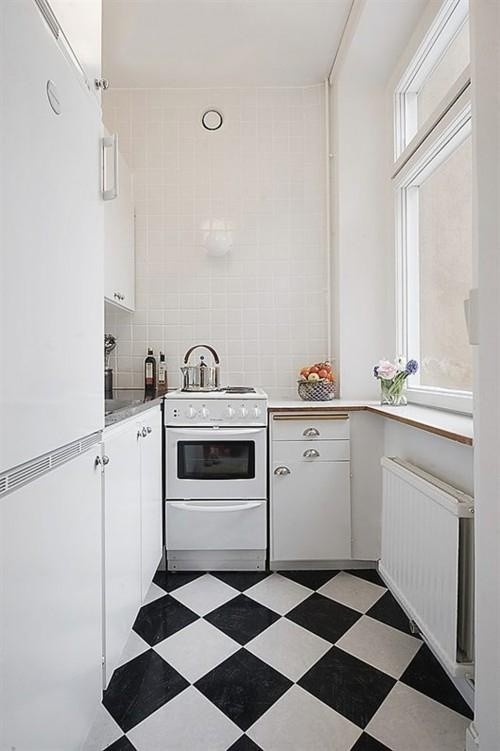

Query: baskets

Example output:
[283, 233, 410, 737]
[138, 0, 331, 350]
[298, 379, 336, 400]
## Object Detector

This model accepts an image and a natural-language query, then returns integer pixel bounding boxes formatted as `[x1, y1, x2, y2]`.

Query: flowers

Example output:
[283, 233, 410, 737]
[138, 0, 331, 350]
[372, 356, 416, 398]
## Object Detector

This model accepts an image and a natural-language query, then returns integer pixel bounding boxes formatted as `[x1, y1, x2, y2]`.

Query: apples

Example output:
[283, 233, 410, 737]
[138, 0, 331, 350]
[300, 361, 331, 381]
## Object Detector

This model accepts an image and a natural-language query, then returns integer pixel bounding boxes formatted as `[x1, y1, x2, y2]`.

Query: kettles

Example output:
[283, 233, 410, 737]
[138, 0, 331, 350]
[180, 343, 221, 392]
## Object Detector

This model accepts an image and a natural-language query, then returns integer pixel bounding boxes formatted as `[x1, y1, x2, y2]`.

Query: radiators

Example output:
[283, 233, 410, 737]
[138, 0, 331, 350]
[376, 457, 474, 683]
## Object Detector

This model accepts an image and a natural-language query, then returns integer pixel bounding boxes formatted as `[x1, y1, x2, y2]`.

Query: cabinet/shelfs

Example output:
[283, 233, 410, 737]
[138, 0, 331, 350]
[1, 0, 118, 751]
[103, 395, 165, 692]
[104, 122, 138, 313]
[269, 416, 354, 569]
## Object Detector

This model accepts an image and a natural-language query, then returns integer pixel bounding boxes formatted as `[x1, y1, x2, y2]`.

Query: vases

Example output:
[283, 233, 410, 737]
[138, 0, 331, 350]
[379, 378, 405, 409]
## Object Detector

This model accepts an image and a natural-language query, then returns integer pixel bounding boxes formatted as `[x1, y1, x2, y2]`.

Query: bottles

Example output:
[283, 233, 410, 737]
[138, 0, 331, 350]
[144, 347, 167, 391]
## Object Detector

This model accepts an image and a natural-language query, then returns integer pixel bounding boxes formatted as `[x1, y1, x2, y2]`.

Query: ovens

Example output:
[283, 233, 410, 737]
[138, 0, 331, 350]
[165, 428, 267, 551]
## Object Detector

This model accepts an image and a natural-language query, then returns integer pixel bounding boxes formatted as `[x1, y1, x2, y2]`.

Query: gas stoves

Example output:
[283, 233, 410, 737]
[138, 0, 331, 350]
[164, 386, 268, 428]
